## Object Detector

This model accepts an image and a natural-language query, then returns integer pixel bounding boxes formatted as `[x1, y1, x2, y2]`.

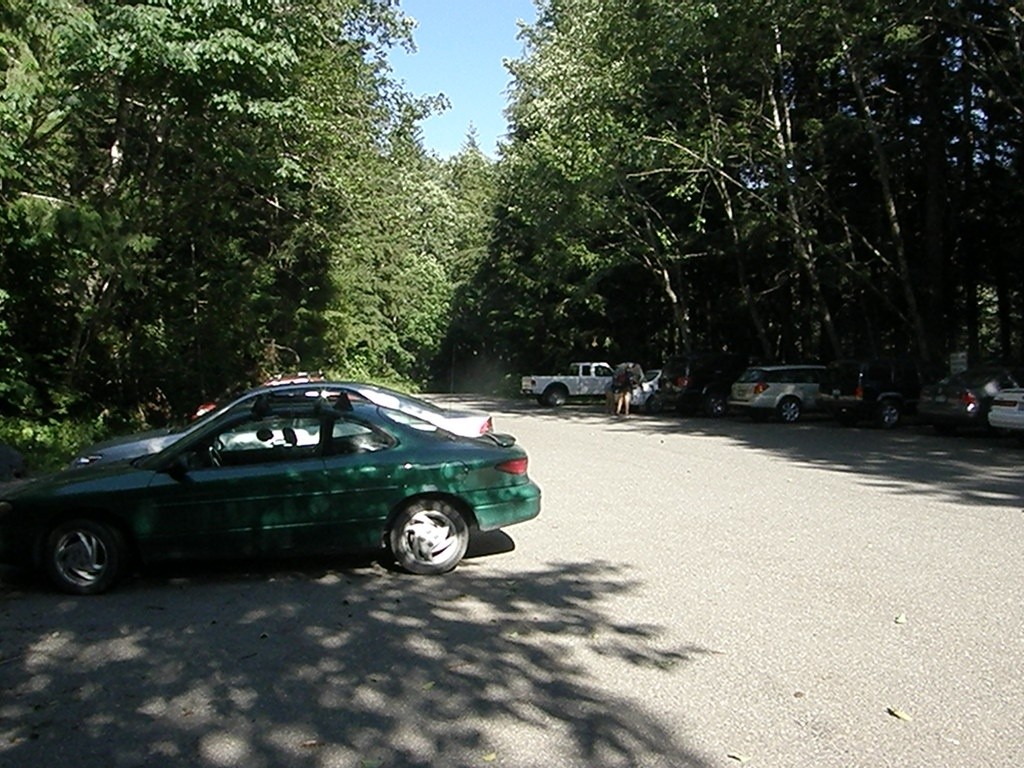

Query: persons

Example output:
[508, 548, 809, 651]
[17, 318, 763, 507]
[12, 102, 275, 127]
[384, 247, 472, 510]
[604, 367, 632, 416]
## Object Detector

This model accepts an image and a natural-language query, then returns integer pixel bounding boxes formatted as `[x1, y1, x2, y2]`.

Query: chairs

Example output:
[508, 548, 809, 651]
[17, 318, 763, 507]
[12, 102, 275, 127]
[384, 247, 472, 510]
[256, 429, 308, 460]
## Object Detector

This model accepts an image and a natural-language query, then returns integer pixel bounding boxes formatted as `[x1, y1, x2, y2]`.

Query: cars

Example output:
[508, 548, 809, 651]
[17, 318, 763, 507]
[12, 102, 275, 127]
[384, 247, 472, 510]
[67, 382, 493, 470]
[604, 369, 664, 415]
[987, 388, 1024, 444]
[726, 365, 826, 423]
[1, 394, 541, 598]
[916, 367, 1024, 438]
[815, 357, 947, 429]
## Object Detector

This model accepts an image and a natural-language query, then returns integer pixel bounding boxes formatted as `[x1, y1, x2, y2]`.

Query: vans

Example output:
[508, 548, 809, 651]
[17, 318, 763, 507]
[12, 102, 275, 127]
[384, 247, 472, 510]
[656, 354, 777, 419]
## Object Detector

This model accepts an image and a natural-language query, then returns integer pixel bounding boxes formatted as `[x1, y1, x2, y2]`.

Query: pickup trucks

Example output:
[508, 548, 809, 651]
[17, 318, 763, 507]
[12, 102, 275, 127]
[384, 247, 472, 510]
[521, 363, 616, 408]
